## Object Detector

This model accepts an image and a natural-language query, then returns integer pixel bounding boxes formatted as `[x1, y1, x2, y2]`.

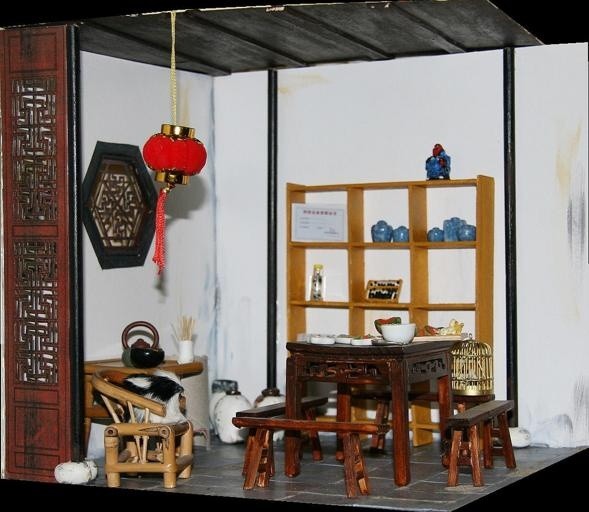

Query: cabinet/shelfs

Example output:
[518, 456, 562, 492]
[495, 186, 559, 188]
[285, 175, 495, 432]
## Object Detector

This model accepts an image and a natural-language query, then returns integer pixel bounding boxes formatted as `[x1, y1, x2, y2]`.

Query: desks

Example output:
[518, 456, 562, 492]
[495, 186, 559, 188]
[283, 339, 462, 485]
[85, 357, 203, 457]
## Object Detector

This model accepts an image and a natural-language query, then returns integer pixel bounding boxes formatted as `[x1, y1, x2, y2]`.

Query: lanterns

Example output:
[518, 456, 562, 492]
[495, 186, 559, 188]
[140, 119, 208, 272]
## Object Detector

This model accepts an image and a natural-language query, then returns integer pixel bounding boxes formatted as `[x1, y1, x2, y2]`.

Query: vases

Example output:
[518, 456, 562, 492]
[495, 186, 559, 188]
[209, 377, 287, 445]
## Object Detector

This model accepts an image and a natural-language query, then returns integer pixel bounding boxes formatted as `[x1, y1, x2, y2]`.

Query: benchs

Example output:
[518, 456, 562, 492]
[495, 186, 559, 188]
[364, 391, 495, 450]
[236, 395, 329, 474]
[446, 400, 516, 487]
[232, 416, 392, 500]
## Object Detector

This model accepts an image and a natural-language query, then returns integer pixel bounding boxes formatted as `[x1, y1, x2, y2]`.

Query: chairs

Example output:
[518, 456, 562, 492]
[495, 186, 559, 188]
[91, 366, 195, 489]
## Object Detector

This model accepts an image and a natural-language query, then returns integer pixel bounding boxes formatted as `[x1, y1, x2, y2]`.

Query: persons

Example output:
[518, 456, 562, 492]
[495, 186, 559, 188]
[425, 143, 453, 181]
